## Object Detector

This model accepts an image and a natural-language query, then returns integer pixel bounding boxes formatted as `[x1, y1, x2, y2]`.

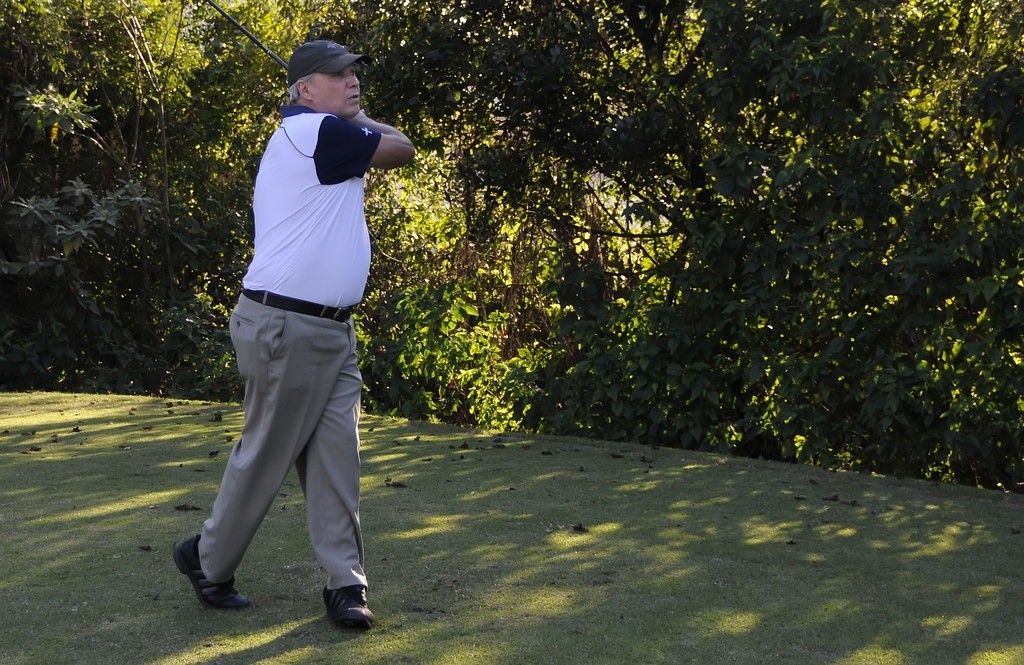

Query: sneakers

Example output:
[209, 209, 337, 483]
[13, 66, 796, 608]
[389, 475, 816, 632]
[175, 532, 251, 609]
[323, 578, 375, 629]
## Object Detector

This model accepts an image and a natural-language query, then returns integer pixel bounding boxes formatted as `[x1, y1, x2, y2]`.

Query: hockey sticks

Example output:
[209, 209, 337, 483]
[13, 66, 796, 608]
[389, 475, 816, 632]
[206, 0, 289, 69]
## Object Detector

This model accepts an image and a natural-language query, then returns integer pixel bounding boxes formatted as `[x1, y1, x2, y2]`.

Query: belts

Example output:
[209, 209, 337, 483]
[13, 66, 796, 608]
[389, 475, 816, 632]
[243, 288, 361, 323]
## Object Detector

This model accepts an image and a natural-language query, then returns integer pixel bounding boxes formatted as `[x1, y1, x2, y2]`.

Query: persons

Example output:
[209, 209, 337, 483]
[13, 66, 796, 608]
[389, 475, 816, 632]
[174, 40, 414, 628]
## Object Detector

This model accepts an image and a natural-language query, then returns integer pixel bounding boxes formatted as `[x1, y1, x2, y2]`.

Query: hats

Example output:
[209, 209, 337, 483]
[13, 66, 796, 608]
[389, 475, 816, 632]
[288, 40, 373, 87]
